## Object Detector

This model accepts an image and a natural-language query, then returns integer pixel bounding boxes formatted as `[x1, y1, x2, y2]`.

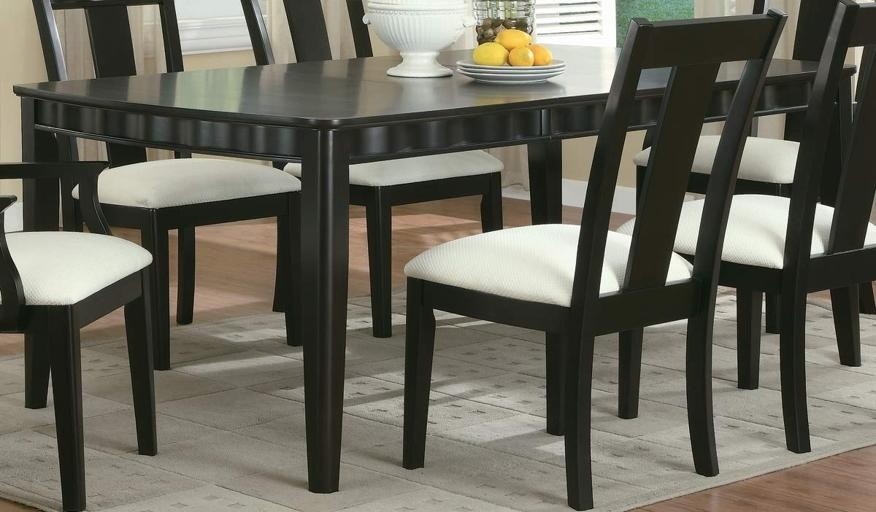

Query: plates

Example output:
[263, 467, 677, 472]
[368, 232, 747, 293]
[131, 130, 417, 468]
[455, 58, 567, 86]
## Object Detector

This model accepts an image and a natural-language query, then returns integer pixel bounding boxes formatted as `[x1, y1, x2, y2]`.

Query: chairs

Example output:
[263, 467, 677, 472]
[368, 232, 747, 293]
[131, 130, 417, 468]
[0, 161, 158, 512]
[633, 0, 876, 333]
[31, 0, 304, 370]
[613, 0, 875, 454]
[402, 0, 788, 511]
[241, 0, 505, 338]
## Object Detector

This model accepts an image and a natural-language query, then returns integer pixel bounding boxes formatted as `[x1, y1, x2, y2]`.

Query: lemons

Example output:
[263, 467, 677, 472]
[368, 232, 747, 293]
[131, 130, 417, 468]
[473, 43, 509, 65]
[531, 43, 552, 66]
[494, 29, 532, 50]
[509, 46, 534, 67]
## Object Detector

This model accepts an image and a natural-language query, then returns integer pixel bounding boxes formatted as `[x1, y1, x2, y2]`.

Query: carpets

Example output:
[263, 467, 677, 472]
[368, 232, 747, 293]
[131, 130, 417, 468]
[0, 282, 876, 511]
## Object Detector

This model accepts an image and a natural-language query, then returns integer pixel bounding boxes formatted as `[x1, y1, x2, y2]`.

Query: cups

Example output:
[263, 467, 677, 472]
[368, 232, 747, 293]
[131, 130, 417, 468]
[472, 1, 538, 47]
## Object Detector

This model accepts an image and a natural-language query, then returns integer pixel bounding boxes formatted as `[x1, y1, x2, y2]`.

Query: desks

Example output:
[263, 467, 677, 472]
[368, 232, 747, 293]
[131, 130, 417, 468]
[12, 45, 856, 494]
